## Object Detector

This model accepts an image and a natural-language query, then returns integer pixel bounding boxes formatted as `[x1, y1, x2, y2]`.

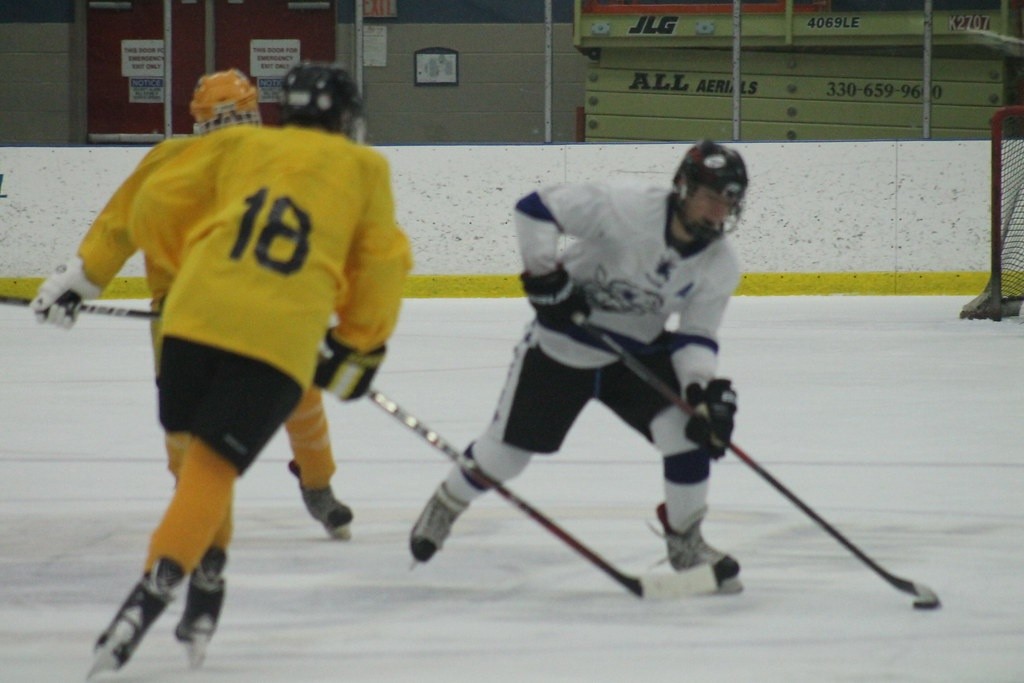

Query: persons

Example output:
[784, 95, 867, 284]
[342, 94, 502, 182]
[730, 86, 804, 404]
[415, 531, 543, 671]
[408, 140, 748, 596]
[30, 60, 415, 683]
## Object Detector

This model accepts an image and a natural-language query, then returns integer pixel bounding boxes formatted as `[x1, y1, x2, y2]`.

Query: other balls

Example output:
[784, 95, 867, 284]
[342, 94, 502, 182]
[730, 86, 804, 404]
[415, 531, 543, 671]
[912, 598, 939, 609]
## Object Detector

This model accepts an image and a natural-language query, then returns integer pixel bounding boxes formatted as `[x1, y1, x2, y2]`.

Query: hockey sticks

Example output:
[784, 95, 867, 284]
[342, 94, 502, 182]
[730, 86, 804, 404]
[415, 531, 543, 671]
[364, 388, 644, 600]
[1, 293, 160, 319]
[571, 310, 943, 611]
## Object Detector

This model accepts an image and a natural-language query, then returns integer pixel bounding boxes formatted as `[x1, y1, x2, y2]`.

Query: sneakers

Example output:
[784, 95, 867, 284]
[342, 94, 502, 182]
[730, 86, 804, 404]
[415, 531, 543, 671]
[175, 547, 227, 666]
[409, 481, 470, 568]
[656, 503, 741, 593]
[84, 556, 184, 680]
[288, 459, 353, 540]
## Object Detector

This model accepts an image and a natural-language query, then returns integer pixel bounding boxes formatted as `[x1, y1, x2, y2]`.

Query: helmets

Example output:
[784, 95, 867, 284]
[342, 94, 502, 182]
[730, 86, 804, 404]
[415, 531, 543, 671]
[282, 61, 366, 129]
[674, 138, 748, 210]
[190, 68, 260, 132]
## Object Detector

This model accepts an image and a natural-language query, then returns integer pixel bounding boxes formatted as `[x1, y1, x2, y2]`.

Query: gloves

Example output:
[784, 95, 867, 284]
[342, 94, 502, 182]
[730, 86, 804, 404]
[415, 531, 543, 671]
[686, 378, 737, 457]
[314, 327, 386, 400]
[521, 262, 591, 330]
[28, 252, 101, 328]
